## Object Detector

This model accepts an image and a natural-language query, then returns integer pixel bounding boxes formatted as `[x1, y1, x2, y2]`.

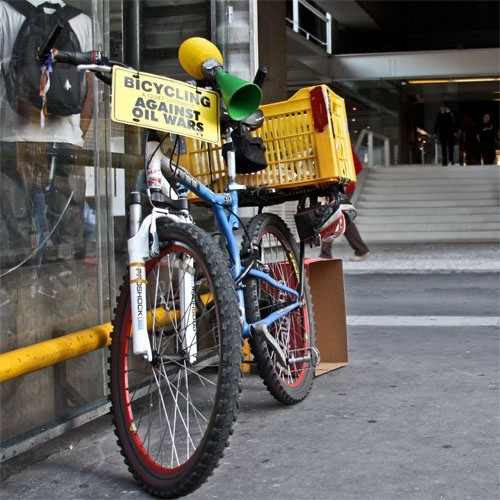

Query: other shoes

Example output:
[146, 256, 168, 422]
[349, 251, 370, 262]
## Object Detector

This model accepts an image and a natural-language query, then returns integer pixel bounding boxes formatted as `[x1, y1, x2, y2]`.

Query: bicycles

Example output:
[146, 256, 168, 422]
[49, 46, 319, 500]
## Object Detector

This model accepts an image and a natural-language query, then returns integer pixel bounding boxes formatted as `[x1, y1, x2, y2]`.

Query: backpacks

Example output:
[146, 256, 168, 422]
[4, 0, 88, 122]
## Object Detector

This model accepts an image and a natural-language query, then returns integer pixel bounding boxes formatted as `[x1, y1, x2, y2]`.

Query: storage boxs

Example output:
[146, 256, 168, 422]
[173, 84, 357, 203]
[261, 257, 349, 378]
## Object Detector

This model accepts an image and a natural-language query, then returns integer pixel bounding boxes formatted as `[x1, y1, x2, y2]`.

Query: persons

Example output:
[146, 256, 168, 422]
[1, 0, 95, 346]
[431, 102, 500, 164]
[29, 168, 93, 307]
[318, 147, 372, 260]
